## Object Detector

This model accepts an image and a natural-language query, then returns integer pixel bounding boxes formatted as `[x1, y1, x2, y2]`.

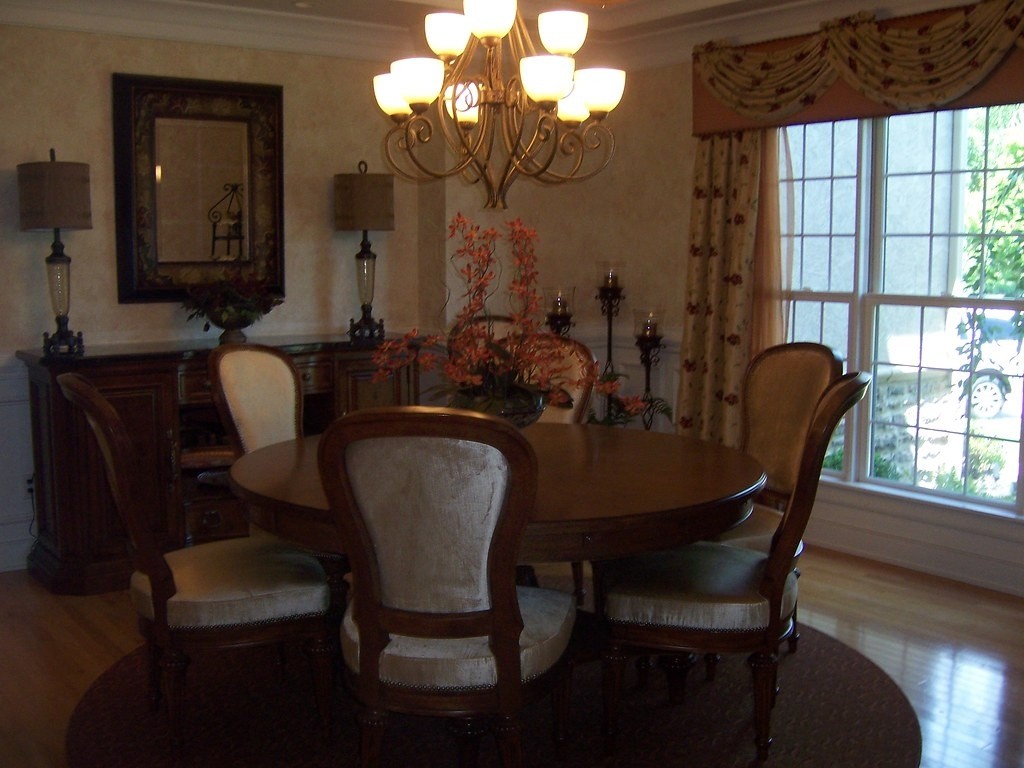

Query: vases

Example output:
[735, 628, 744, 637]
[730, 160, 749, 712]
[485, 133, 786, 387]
[449, 370, 549, 429]
[207, 312, 251, 346]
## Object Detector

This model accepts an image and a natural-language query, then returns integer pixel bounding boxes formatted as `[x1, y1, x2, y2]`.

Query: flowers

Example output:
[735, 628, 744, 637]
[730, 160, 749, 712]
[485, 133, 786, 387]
[364, 210, 673, 433]
[179, 271, 283, 332]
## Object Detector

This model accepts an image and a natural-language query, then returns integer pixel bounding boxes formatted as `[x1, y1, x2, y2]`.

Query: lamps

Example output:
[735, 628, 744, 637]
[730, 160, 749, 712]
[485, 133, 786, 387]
[17, 148, 92, 355]
[373, 0, 629, 212]
[332, 160, 397, 343]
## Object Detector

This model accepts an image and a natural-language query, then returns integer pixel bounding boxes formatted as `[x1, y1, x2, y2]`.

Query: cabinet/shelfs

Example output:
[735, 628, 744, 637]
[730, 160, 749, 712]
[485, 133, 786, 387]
[13, 331, 422, 597]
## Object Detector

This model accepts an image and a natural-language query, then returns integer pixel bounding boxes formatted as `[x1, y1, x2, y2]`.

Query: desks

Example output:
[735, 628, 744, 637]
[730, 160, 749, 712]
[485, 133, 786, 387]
[226, 417, 769, 668]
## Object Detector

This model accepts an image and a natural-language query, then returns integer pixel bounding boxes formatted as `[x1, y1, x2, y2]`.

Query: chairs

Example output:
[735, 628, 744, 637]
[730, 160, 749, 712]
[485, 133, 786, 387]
[207, 343, 306, 666]
[599, 341, 866, 763]
[314, 405, 578, 768]
[54, 369, 342, 768]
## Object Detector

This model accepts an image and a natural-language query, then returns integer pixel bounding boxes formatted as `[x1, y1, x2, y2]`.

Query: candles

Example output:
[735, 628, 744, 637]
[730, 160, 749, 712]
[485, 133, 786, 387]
[551, 289, 566, 315]
[604, 272, 617, 288]
[642, 311, 658, 337]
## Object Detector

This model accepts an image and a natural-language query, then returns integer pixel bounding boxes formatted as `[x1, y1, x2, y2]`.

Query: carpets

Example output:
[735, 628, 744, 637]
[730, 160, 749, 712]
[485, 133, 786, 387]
[66, 572, 920, 768]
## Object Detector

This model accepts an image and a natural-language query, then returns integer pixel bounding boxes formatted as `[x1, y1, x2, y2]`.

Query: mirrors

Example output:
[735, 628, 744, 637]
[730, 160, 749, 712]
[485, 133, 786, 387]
[111, 71, 285, 306]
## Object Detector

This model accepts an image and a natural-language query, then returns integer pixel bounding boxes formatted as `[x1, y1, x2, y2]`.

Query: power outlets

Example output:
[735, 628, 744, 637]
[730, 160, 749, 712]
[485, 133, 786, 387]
[23, 472, 36, 503]
